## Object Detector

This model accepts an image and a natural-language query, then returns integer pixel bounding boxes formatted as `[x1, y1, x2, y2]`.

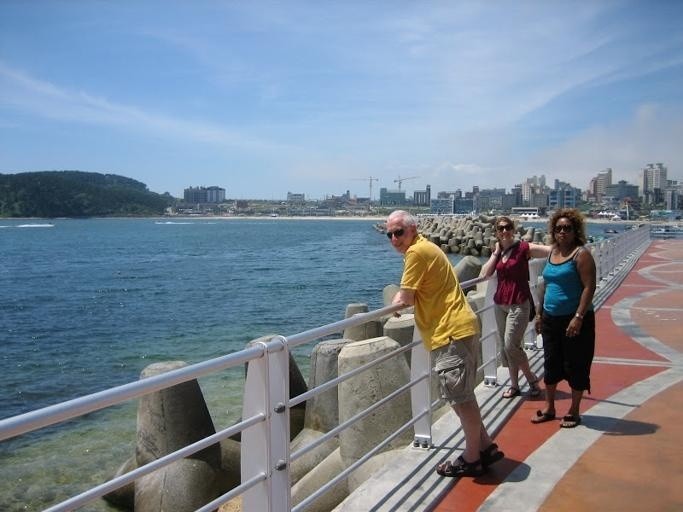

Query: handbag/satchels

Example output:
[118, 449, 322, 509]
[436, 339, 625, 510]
[529, 293, 536, 321]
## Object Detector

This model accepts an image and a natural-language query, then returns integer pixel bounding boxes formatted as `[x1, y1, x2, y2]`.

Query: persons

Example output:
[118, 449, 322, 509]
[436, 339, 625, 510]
[383, 210, 505, 477]
[530, 208, 598, 429]
[477, 215, 554, 399]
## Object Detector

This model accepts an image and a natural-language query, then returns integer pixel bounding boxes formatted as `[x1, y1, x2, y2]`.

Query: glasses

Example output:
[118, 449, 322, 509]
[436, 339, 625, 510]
[553, 225, 572, 233]
[386, 228, 404, 239]
[497, 225, 512, 231]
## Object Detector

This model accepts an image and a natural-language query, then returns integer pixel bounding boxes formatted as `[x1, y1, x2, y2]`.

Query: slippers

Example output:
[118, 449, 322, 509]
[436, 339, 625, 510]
[531, 410, 555, 423]
[560, 416, 580, 428]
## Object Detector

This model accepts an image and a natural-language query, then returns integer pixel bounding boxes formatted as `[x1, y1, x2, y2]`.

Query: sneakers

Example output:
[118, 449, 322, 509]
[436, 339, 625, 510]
[502, 385, 520, 398]
[529, 374, 540, 396]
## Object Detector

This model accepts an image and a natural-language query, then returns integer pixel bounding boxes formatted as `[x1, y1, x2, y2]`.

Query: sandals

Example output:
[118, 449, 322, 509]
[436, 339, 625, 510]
[437, 455, 486, 477]
[479, 443, 504, 465]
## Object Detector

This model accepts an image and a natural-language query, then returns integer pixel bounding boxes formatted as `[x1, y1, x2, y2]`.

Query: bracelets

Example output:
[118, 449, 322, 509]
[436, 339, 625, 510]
[534, 314, 541, 321]
[490, 251, 499, 256]
[573, 312, 584, 320]
